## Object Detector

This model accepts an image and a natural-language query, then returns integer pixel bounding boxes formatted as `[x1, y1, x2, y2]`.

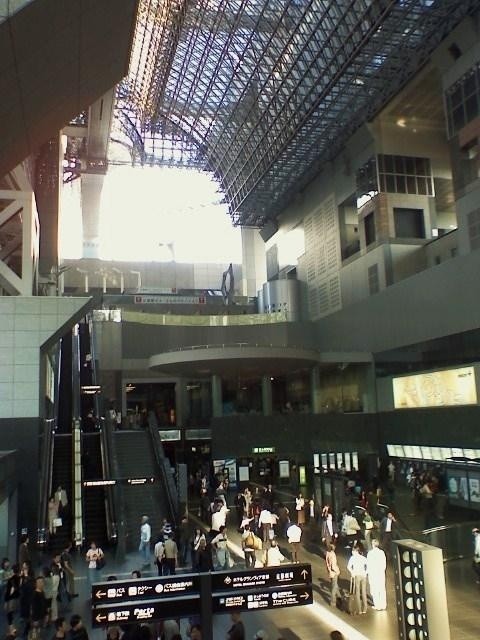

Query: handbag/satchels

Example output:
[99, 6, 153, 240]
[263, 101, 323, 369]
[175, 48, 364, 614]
[246, 532, 262, 550]
[96, 557, 106, 569]
[365, 522, 373, 529]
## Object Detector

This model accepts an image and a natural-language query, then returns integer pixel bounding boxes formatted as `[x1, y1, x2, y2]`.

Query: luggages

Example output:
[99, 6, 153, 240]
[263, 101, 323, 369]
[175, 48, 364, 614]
[342, 575, 357, 616]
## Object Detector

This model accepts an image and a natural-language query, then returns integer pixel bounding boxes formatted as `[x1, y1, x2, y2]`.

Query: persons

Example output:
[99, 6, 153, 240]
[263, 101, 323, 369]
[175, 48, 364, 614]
[0, 464, 446, 640]
[471, 528, 480, 584]
